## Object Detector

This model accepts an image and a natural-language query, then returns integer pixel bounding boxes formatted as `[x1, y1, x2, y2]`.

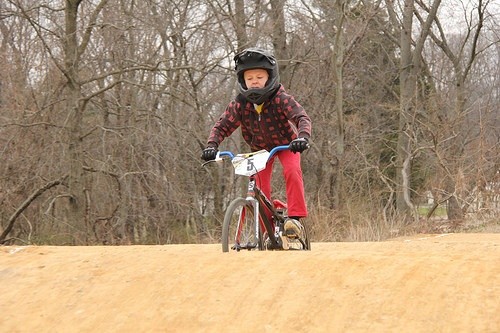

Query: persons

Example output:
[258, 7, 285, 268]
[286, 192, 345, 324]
[202, 47, 312, 239]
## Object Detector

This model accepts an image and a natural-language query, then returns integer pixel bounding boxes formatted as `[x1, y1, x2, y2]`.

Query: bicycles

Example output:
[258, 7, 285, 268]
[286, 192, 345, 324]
[202, 143, 312, 252]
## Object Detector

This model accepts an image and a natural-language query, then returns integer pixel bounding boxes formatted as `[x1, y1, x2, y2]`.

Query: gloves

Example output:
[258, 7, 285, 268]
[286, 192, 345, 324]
[202, 146, 217, 161]
[290, 137, 307, 154]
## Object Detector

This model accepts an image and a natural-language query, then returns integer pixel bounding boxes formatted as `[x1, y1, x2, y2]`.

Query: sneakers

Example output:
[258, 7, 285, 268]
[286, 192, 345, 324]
[264, 232, 283, 247]
[284, 219, 303, 238]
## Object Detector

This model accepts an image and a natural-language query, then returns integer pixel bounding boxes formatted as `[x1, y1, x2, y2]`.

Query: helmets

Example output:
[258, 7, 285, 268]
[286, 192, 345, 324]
[233, 47, 280, 103]
